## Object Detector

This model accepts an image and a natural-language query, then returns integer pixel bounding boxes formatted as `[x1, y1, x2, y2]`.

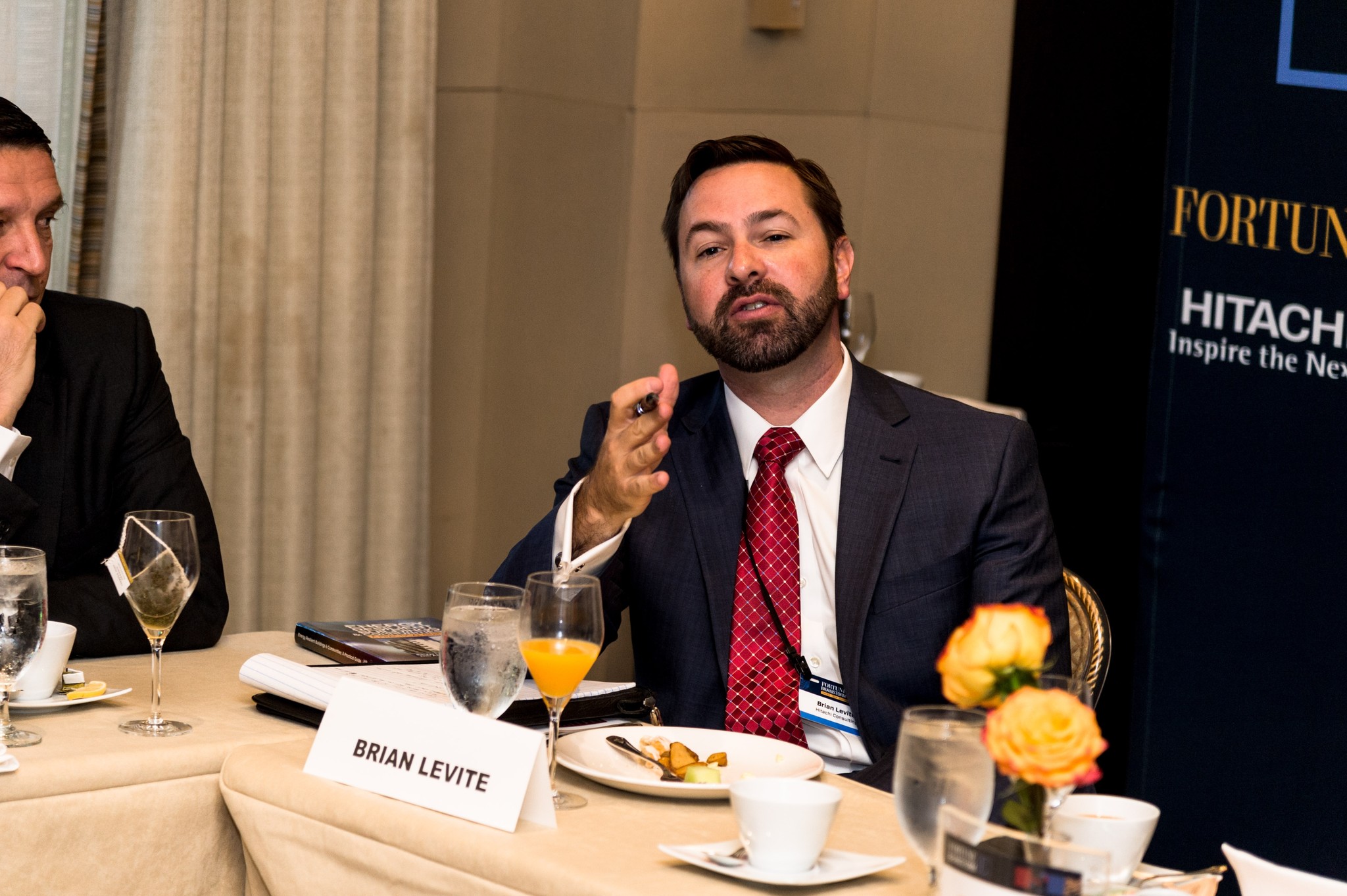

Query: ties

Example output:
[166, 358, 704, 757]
[725, 427, 808, 748]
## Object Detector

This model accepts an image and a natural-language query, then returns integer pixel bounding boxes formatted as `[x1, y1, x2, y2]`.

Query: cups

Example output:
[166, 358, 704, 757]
[839, 289, 877, 366]
[729, 778, 844, 875]
[438, 580, 531, 725]
[1049, 794, 1162, 884]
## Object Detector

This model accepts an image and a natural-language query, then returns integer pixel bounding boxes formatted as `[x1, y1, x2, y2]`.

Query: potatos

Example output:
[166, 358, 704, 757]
[657, 742, 727, 779]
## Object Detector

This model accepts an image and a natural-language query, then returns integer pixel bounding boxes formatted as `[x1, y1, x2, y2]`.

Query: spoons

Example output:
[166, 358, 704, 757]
[1124, 856, 1228, 890]
[701, 844, 748, 868]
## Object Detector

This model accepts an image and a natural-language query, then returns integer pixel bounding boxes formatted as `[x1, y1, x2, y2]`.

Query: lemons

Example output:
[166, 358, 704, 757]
[67, 681, 107, 701]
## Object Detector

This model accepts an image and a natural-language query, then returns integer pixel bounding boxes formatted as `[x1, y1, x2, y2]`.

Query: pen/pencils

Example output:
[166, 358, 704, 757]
[634, 392, 660, 417]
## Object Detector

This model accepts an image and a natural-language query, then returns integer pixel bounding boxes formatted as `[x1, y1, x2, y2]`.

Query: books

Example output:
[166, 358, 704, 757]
[295, 616, 442, 663]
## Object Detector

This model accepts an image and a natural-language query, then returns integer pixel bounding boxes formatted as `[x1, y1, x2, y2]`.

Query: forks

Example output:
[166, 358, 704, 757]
[607, 734, 682, 784]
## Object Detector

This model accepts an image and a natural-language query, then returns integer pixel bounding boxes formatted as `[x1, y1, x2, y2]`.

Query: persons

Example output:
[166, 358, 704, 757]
[0, 93, 232, 661]
[489, 137, 1070, 837]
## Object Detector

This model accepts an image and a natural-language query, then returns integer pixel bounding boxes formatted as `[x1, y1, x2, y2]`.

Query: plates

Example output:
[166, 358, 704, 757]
[6, 683, 134, 717]
[657, 835, 908, 890]
[550, 723, 826, 803]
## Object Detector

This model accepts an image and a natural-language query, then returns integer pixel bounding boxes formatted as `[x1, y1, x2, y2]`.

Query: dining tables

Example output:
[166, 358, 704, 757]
[0, 632, 340, 896]
[218, 716, 1148, 896]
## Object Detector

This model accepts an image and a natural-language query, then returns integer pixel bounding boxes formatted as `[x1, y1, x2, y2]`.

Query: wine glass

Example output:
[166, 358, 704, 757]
[118, 508, 199, 740]
[894, 702, 998, 896]
[0, 540, 48, 749]
[515, 570, 604, 811]
